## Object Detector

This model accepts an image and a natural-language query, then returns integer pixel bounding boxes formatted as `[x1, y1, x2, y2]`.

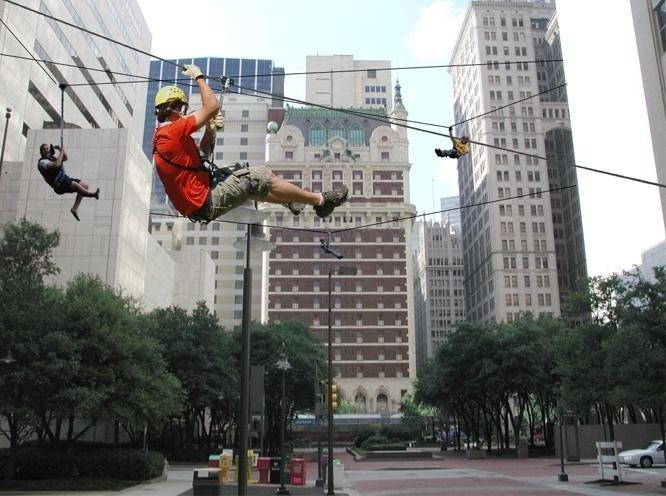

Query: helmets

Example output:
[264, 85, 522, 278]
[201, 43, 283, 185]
[154, 85, 192, 110]
[461, 135, 471, 141]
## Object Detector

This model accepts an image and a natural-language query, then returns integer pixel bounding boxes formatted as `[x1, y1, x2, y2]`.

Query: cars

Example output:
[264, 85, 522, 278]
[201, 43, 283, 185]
[618, 440, 666, 468]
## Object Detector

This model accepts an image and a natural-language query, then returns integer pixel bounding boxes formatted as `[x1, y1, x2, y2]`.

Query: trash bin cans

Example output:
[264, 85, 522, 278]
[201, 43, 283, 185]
[193, 469, 221, 496]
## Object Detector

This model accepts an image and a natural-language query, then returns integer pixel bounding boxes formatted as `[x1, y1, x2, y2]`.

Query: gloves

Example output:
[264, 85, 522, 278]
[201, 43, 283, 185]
[180, 63, 205, 81]
[205, 111, 225, 135]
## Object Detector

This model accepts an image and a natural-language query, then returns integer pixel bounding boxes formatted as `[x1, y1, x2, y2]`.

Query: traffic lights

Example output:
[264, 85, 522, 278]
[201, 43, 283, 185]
[332, 385, 338, 412]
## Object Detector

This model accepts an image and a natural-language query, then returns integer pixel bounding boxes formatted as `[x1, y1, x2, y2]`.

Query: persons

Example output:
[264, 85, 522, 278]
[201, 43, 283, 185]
[435, 125, 472, 159]
[153, 64, 348, 226]
[37, 142, 98, 222]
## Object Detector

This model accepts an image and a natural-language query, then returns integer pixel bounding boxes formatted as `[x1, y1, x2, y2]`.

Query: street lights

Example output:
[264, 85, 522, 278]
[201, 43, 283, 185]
[328, 268, 345, 496]
[555, 405, 568, 481]
[232, 223, 277, 496]
[275, 353, 292, 495]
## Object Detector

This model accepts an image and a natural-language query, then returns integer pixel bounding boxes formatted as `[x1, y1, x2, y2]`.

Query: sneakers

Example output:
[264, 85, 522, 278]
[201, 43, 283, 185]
[288, 196, 306, 216]
[70, 185, 101, 221]
[315, 184, 348, 219]
[435, 148, 442, 158]
